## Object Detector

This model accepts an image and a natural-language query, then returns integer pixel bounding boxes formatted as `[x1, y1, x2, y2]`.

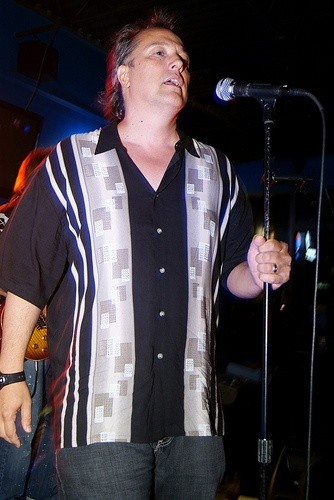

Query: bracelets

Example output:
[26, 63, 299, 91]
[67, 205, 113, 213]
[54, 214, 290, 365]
[0, 370, 27, 389]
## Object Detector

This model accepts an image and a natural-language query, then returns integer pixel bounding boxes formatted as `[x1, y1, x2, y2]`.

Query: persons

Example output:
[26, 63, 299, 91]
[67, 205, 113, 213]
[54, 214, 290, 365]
[1, 14, 292, 499]
[0, 146, 59, 500]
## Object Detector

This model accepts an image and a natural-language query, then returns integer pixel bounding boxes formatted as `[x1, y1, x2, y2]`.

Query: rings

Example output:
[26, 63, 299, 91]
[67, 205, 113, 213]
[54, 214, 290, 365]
[272, 263, 277, 273]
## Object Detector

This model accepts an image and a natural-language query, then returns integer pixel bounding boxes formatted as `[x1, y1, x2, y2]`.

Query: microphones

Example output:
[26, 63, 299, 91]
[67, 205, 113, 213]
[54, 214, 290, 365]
[215, 78, 310, 105]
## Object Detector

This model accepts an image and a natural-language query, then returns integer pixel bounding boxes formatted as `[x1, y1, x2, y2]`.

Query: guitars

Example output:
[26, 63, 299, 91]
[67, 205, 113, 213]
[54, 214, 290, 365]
[0, 296, 50, 361]
